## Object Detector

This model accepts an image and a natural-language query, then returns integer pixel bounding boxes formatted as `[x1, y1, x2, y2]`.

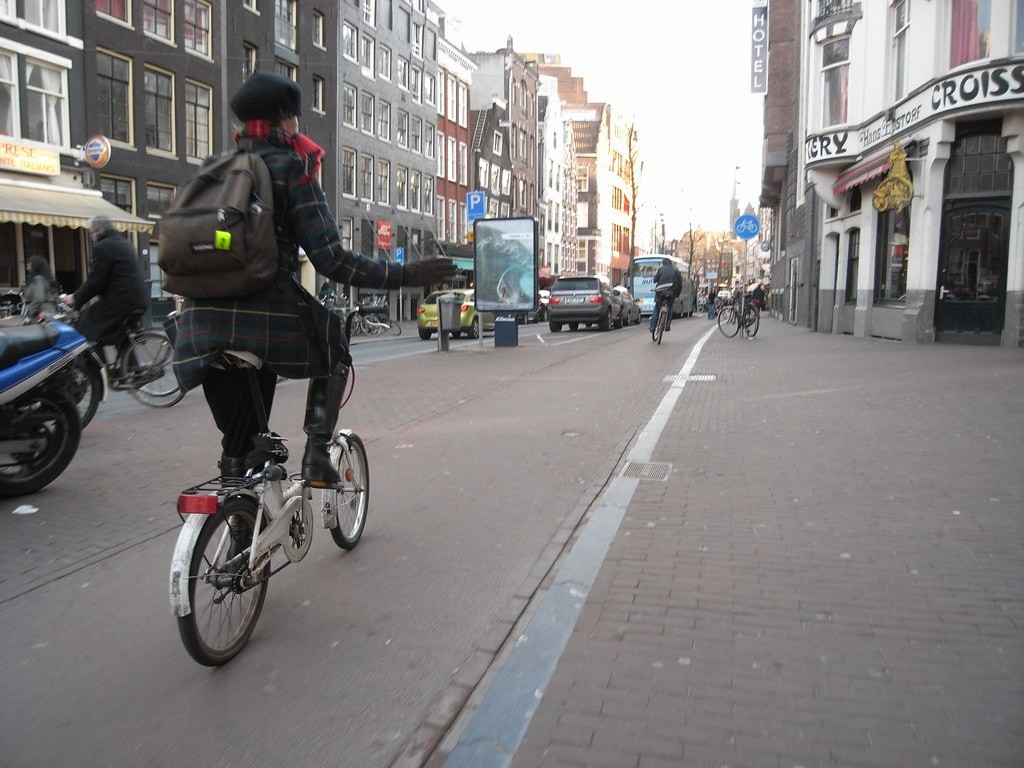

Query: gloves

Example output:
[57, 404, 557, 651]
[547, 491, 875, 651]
[403, 258, 457, 287]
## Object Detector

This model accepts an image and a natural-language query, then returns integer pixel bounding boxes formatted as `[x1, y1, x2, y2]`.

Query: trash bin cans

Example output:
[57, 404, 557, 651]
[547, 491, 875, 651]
[437, 291, 465, 331]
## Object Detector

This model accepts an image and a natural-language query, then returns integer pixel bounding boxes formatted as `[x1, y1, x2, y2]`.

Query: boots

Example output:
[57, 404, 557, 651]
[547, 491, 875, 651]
[218, 450, 261, 563]
[302, 362, 349, 483]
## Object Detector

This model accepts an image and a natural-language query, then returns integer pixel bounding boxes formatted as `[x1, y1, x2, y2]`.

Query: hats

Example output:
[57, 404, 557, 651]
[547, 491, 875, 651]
[230, 72, 302, 125]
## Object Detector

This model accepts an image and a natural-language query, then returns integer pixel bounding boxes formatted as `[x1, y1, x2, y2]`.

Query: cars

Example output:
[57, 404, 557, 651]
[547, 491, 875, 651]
[718, 290, 733, 306]
[417, 288, 496, 340]
[613, 285, 641, 329]
[512, 289, 550, 325]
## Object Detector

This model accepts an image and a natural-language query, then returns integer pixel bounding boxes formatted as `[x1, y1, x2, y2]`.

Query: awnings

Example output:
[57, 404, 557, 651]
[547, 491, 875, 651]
[437, 255, 474, 271]
[1, 179, 156, 234]
[832, 139, 915, 194]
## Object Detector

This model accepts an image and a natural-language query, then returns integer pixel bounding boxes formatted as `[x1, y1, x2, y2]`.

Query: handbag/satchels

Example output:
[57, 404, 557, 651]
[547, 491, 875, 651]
[655, 283, 674, 302]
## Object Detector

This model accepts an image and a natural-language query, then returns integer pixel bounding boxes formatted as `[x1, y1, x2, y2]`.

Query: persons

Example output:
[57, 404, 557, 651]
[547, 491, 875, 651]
[61, 215, 150, 367]
[319, 282, 336, 300]
[172, 74, 457, 561]
[22, 256, 58, 325]
[708, 284, 764, 319]
[648, 258, 682, 332]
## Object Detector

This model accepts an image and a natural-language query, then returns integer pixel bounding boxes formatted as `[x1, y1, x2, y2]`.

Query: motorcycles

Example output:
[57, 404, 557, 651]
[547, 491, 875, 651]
[0, 320, 99, 499]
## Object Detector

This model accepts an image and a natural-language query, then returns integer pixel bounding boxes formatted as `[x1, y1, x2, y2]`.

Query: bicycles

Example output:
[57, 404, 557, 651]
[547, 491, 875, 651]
[3, 287, 186, 408]
[310, 287, 402, 338]
[652, 280, 671, 345]
[169, 294, 389, 667]
[718, 283, 760, 338]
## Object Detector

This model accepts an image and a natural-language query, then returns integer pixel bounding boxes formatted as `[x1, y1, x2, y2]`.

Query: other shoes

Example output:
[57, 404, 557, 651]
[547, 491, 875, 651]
[648, 325, 654, 332]
[665, 325, 670, 331]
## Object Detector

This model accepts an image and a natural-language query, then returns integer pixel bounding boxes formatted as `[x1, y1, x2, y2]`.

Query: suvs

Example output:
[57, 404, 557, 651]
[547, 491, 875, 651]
[547, 271, 621, 331]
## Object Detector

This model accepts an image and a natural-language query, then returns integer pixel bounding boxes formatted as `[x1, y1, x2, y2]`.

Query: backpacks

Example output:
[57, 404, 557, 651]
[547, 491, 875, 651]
[158, 144, 289, 300]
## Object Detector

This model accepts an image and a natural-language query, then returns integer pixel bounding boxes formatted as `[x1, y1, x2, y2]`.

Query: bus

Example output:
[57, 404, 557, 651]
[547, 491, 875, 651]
[624, 253, 700, 319]
